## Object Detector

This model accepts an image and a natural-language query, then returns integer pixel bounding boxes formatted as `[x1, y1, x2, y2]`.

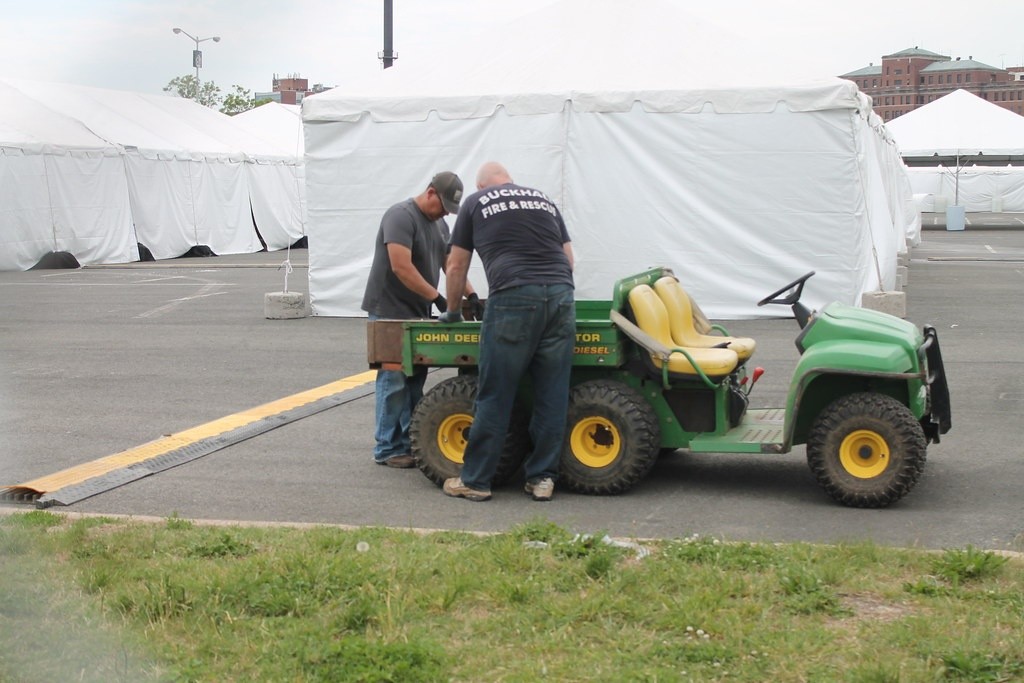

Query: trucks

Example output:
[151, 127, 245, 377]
[364, 265, 951, 508]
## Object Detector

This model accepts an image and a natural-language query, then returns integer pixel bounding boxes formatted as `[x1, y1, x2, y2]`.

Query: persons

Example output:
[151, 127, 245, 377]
[361, 172, 485, 467]
[439, 161, 575, 502]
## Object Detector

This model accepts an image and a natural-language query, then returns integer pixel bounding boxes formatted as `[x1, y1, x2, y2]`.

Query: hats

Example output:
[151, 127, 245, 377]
[432, 171, 464, 214]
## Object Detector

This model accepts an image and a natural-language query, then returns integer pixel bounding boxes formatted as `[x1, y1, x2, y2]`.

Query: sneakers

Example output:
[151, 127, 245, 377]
[524, 477, 555, 501]
[376, 455, 416, 468]
[443, 477, 492, 501]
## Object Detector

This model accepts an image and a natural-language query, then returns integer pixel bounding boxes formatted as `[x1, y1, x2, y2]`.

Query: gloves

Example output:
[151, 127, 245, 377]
[432, 294, 447, 313]
[438, 310, 465, 323]
[467, 293, 485, 320]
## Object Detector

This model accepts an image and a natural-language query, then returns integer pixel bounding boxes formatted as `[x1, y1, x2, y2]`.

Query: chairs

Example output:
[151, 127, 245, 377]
[626, 276, 756, 383]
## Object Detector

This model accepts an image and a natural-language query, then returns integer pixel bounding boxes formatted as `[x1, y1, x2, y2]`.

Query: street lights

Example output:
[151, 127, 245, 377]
[172, 27, 220, 104]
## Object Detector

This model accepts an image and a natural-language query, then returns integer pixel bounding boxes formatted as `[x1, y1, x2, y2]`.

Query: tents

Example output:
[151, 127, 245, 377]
[302, 0, 1024, 318]
[0, 77, 304, 271]
